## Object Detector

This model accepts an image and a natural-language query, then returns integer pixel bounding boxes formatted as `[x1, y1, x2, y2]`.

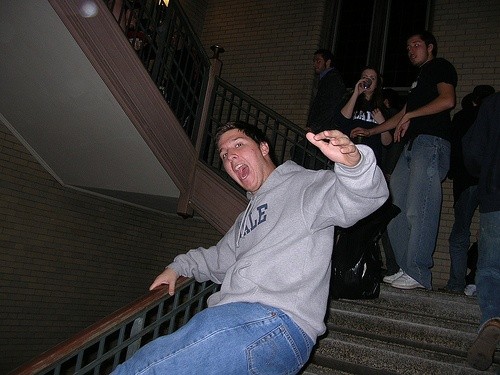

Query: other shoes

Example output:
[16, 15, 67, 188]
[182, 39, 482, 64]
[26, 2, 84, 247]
[383, 268, 427, 289]
[465, 320, 500, 371]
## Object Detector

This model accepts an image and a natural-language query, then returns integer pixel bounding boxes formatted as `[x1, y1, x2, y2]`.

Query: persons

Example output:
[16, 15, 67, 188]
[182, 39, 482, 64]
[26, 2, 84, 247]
[108, 119, 389, 375]
[449, 84, 500, 369]
[290, 48, 351, 174]
[371, 87, 401, 275]
[350, 29, 457, 290]
[327, 70, 395, 269]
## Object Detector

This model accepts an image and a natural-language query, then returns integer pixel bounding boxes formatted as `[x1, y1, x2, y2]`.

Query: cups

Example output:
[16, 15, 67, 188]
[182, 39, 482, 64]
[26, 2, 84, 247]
[363, 77, 372, 89]
